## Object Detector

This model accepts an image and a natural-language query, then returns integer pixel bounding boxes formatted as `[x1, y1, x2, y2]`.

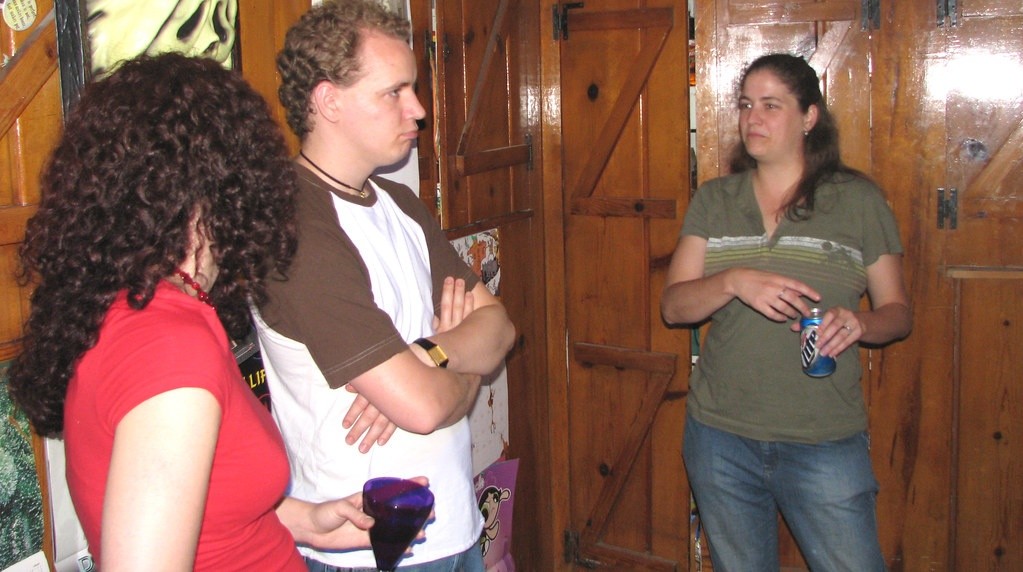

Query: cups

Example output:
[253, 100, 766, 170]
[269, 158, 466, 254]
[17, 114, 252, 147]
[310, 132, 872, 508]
[362, 477, 434, 572]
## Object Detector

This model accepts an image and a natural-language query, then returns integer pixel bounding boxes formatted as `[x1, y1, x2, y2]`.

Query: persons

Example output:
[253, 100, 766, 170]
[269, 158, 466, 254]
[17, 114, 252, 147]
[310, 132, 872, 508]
[6, 52, 437, 572]
[247, 0, 519, 571]
[659, 52, 914, 572]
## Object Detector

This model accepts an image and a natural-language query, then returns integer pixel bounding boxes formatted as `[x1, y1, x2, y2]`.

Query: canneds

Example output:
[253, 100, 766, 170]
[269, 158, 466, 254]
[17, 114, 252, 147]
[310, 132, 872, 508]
[799, 308, 836, 378]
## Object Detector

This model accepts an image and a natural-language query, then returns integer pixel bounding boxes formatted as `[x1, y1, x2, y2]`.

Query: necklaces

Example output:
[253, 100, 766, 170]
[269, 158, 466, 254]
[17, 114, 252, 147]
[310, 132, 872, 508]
[168, 262, 218, 316]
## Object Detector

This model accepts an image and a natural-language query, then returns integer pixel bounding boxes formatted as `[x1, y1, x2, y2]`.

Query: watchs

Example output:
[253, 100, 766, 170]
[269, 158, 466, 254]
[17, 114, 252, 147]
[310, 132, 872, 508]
[413, 337, 448, 370]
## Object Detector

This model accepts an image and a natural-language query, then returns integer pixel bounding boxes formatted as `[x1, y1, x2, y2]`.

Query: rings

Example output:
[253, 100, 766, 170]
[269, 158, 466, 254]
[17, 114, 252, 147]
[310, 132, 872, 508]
[841, 325, 851, 336]
